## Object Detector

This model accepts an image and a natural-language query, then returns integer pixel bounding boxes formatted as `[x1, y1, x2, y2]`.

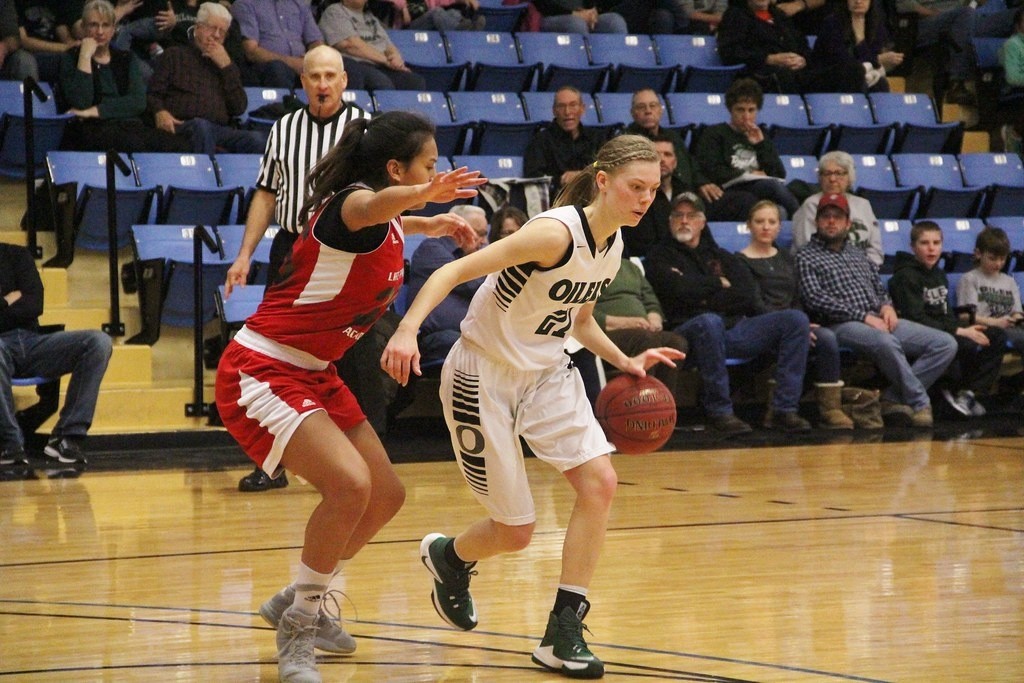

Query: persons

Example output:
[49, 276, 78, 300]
[208, 109, 487, 682]
[0, 0, 1023, 441]
[379, 133, 688, 681]
[219, 45, 377, 496]
[0, 233, 115, 477]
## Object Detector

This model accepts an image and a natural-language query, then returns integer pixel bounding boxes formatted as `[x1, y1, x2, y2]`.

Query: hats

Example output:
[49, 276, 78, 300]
[672, 192, 707, 211]
[817, 192, 853, 215]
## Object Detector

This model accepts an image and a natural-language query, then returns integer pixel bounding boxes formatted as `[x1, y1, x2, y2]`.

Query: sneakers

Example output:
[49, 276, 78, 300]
[419, 532, 478, 631]
[0, 444, 29, 466]
[259, 586, 361, 653]
[530, 607, 605, 679]
[44, 436, 89, 466]
[274, 600, 322, 683]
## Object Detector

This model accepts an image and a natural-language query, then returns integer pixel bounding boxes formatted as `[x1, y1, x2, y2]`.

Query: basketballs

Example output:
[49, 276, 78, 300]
[594, 371, 677, 454]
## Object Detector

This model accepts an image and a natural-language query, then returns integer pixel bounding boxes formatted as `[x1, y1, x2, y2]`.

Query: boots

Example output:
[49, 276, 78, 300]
[819, 380, 851, 428]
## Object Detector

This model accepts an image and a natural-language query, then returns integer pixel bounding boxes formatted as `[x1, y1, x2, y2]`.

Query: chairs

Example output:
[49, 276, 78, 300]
[403, 231, 430, 262]
[522, 91, 623, 149]
[10, 323, 66, 434]
[372, 90, 473, 157]
[239, 86, 291, 133]
[595, 34, 1024, 367]
[0, 79, 76, 182]
[385, 29, 472, 92]
[583, 33, 684, 95]
[119, 225, 234, 348]
[451, 156, 524, 205]
[216, 226, 280, 287]
[212, 154, 264, 224]
[515, 31, 612, 91]
[203, 284, 265, 426]
[295, 89, 382, 118]
[443, 30, 544, 92]
[132, 153, 239, 224]
[22, 151, 165, 268]
[447, 92, 546, 156]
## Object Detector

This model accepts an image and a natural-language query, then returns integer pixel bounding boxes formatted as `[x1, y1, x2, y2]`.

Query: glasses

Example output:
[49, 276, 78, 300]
[670, 213, 703, 221]
[819, 170, 848, 180]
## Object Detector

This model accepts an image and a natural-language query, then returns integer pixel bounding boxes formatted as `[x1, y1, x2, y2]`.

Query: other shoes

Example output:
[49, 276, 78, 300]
[765, 408, 810, 434]
[882, 404, 914, 428]
[703, 412, 754, 434]
[239, 467, 289, 491]
[945, 389, 987, 420]
[914, 407, 934, 427]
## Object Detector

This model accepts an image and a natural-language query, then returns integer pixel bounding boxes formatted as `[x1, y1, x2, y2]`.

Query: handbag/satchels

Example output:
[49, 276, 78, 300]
[842, 383, 884, 429]
